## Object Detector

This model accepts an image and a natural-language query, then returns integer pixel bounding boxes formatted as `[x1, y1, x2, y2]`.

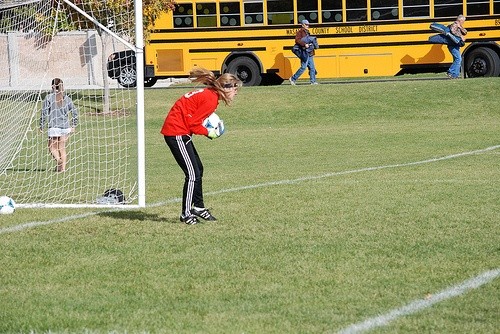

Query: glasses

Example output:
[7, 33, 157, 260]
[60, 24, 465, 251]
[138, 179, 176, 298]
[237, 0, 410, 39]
[305, 24, 309, 26]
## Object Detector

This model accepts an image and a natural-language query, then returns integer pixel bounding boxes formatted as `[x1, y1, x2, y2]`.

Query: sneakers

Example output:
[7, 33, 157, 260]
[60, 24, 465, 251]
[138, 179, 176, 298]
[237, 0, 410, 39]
[310, 82, 318, 85]
[445, 72, 453, 81]
[54, 160, 63, 171]
[179, 213, 204, 226]
[191, 208, 218, 223]
[288, 77, 296, 86]
[451, 75, 462, 79]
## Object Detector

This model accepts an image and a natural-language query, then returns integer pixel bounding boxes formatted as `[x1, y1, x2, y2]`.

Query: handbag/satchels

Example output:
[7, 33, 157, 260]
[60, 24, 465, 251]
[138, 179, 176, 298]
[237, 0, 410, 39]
[427, 33, 465, 47]
[429, 22, 461, 44]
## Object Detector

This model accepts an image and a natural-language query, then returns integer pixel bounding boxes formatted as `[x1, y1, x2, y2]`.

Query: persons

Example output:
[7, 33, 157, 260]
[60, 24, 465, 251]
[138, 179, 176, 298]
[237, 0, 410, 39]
[39, 78, 78, 171]
[446, 14, 467, 80]
[288, 20, 318, 85]
[160, 65, 242, 225]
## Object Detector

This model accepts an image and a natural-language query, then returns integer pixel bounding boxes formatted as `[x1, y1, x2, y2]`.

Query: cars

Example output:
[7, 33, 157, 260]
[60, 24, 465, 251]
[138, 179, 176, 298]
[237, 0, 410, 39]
[107, 47, 157, 88]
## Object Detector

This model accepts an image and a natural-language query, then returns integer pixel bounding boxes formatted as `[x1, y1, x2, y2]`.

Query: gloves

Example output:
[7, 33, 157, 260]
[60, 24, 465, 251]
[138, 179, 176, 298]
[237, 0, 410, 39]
[206, 128, 218, 139]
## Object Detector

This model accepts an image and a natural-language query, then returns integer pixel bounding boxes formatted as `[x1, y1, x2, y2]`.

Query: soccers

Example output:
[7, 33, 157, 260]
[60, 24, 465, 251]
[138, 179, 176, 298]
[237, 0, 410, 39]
[0, 196, 16, 215]
[203, 117, 225, 140]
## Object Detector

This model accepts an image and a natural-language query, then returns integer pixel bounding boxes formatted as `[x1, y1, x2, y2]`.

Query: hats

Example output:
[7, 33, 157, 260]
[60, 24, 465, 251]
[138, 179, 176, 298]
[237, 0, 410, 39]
[301, 20, 310, 24]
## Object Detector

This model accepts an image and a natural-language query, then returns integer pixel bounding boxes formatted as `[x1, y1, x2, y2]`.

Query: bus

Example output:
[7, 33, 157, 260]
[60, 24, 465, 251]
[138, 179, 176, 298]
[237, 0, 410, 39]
[144, 0, 500, 86]
